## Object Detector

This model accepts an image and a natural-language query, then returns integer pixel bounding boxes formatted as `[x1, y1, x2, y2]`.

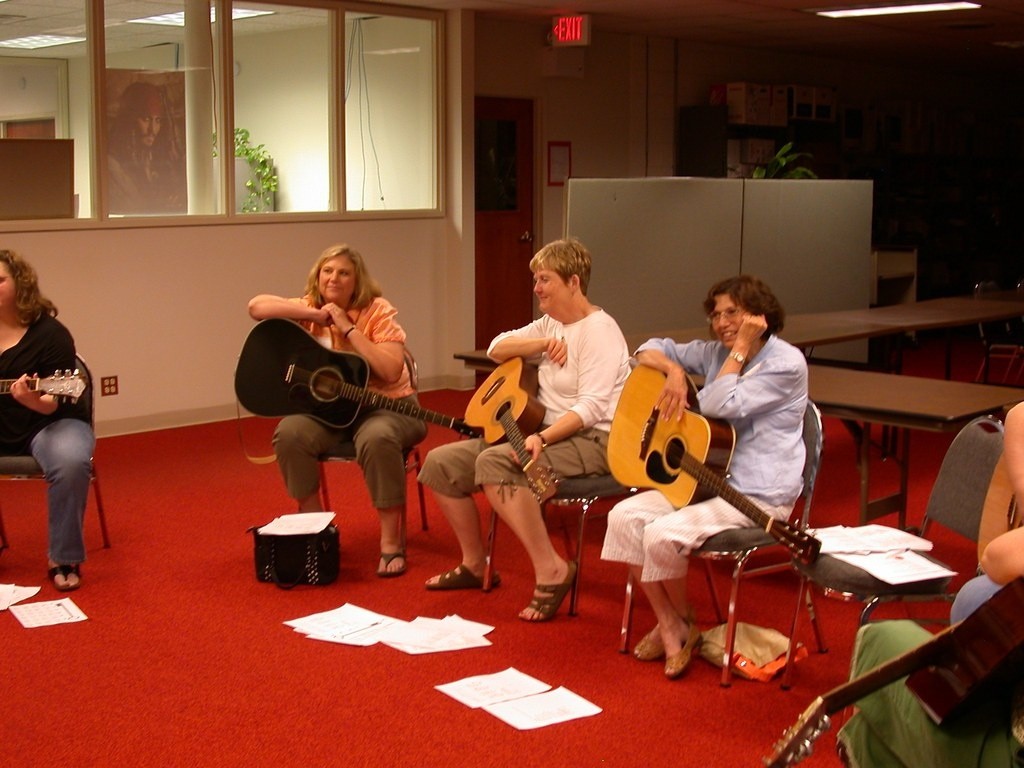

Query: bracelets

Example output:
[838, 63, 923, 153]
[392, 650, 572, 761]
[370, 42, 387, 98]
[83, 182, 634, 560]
[729, 351, 746, 365]
[345, 325, 356, 337]
[534, 431, 549, 450]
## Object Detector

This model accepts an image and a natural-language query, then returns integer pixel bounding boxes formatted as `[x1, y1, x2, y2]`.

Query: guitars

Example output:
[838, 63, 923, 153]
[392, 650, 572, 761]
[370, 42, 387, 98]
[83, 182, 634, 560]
[232, 316, 484, 440]
[0, 368, 89, 406]
[605, 363, 823, 567]
[761, 570, 1024, 768]
[463, 355, 562, 506]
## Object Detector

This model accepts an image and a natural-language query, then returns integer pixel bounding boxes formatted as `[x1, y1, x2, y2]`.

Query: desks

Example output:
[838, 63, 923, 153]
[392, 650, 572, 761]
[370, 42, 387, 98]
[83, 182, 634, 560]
[688, 363, 1024, 530]
[454, 285, 1024, 470]
[872, 243, 922, 345]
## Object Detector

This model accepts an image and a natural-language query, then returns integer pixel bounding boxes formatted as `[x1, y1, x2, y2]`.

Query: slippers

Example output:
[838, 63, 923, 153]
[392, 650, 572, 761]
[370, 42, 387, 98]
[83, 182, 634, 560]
[376, 553, 406, 576]
[425, 564, 500, 590]
[521, 561, 577, 622]
[50, 565, 82, 590]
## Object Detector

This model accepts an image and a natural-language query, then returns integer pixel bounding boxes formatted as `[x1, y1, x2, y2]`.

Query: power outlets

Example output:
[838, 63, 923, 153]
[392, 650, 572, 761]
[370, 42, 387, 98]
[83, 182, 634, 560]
[101, 376, 120, 397]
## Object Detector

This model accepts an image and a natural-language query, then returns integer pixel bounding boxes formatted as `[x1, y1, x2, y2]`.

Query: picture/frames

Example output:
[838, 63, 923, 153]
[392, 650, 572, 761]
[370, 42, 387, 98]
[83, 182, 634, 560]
[546, 140, 571, 188]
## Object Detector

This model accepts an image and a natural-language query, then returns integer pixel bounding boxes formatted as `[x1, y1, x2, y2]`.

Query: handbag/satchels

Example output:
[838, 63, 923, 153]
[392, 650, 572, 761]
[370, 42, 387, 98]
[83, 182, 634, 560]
[247, 523, 338, 589]
[696, 622, 807, 683]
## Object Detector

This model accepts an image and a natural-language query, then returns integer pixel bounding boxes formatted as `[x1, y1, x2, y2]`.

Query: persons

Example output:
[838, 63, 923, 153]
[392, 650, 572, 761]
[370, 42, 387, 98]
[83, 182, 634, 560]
[418, 234, 631, 622]
[598, 278, 808, 678]
[246, 245, 427, 574]
[838, 396, 1024, 768]
[0, 248, 93, 593]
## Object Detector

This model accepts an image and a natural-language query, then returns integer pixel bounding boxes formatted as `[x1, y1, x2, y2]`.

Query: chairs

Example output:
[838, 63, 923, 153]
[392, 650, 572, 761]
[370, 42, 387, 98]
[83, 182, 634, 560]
[974, 278, 1024, 383]
[475, 411, 637, 615]
[0, 353, 112, 551]
[300, 345, 428, 534]
[777, 417, 1014, 693]
[617, 396, 825, 687]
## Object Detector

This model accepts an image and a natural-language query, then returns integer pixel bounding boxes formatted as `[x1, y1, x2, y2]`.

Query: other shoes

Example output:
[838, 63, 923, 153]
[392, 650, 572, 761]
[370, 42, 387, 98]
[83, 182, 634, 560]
[665, 623, 702, 677]
[634, 632, 665, 660]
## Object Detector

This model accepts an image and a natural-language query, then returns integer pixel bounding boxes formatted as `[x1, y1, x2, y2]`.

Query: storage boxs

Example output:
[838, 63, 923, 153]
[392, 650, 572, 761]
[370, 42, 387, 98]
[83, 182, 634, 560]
[700, 75, 1024, 173]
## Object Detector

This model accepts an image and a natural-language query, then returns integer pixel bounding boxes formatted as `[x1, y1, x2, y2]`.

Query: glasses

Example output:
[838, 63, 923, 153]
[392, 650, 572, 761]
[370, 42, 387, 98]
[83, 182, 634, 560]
[706, 307, 745, 323]
